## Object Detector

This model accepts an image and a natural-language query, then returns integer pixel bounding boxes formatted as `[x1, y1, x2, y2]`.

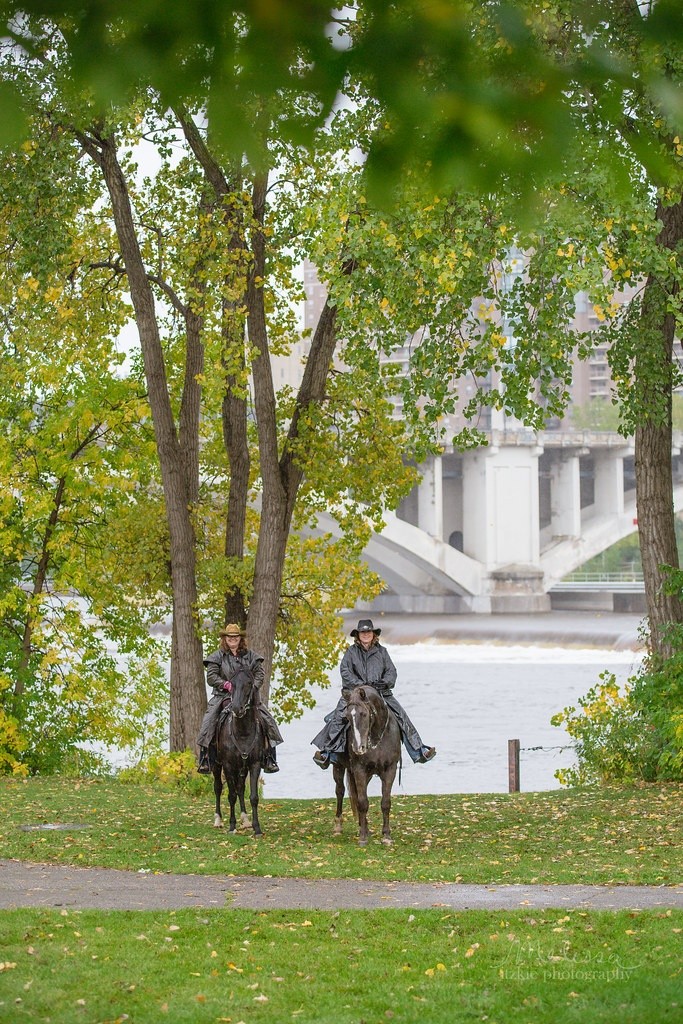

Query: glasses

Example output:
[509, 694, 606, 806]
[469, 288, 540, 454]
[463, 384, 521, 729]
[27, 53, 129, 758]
[225, 634, 240, 638]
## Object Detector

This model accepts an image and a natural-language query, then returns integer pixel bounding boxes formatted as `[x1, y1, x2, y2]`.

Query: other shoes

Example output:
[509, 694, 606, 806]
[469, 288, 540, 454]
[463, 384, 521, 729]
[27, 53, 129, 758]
[419, 747, 435, 763]
[264, 752, 279, 773]
[315, 751, 338, 765]
[199, 746, 209, 772]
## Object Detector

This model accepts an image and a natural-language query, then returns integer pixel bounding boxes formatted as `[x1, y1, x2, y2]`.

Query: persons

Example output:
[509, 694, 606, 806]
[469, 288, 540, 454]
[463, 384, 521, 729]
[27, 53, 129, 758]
[310, 619, 435, 769]
[195, 624, 284, 774]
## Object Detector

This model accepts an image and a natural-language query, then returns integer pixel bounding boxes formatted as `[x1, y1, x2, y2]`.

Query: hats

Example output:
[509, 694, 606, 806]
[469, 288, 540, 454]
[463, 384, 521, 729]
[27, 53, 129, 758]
[219, 624, 246, 638]
[350, 620, 381, 637]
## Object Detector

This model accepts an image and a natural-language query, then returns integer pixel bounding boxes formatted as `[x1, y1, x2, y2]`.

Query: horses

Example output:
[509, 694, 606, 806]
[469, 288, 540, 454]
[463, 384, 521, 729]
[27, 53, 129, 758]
[207, 657, 267, 837]
[330, 684, 402, 847]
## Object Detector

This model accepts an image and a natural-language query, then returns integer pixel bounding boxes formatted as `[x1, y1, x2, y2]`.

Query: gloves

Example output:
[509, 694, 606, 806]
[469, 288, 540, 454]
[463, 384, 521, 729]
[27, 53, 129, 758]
[373, 680, 388, 691]
[223, 680, 233, 694]
